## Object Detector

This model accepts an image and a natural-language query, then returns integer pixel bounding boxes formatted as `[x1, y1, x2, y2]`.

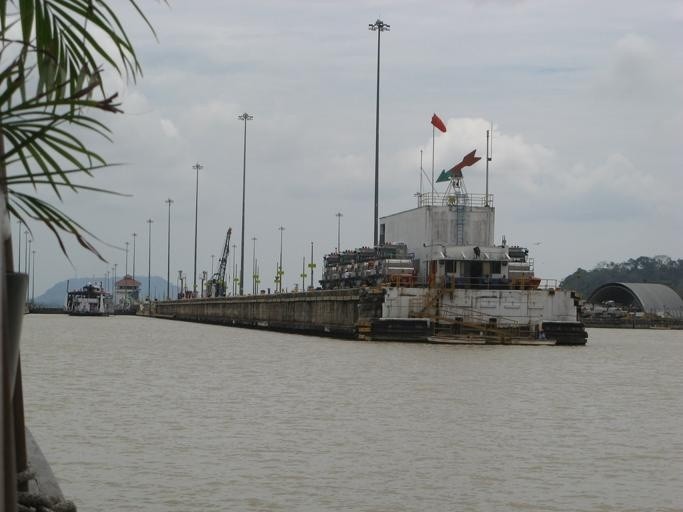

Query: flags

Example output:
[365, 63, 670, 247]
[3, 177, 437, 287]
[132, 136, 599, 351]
[430, 112, 448, 132]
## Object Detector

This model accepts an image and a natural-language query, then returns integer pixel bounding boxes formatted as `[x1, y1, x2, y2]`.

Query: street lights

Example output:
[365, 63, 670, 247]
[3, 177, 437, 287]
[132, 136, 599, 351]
[368, 20, 390, 245]
[105, 162, 202, 298]
[211, 112, 342, 295]
[17, 220, 36, 306]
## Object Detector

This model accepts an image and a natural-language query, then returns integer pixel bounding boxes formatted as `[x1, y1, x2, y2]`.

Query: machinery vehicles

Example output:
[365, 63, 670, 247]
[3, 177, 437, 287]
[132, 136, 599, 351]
[207, 227, 232, 296]
